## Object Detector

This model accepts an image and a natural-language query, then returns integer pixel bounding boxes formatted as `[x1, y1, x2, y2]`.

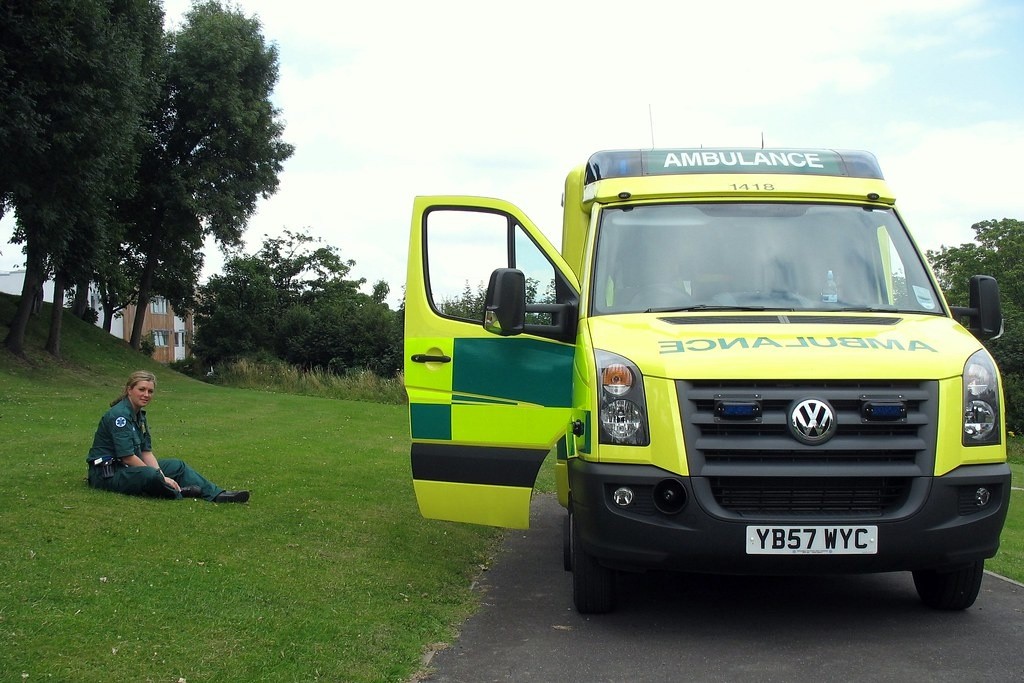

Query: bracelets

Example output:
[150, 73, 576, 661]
[158, 468, 161, 471]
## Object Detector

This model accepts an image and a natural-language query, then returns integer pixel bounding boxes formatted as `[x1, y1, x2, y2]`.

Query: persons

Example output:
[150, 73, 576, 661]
[86, 370, 249, 504]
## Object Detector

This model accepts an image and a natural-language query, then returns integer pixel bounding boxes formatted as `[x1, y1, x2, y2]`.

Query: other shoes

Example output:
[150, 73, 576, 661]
[215, 490, 250, 502]
[179, 485, 202, 498]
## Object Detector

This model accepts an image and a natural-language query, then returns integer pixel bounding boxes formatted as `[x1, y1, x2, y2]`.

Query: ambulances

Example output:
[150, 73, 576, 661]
[402, 147, 1010, 615]
